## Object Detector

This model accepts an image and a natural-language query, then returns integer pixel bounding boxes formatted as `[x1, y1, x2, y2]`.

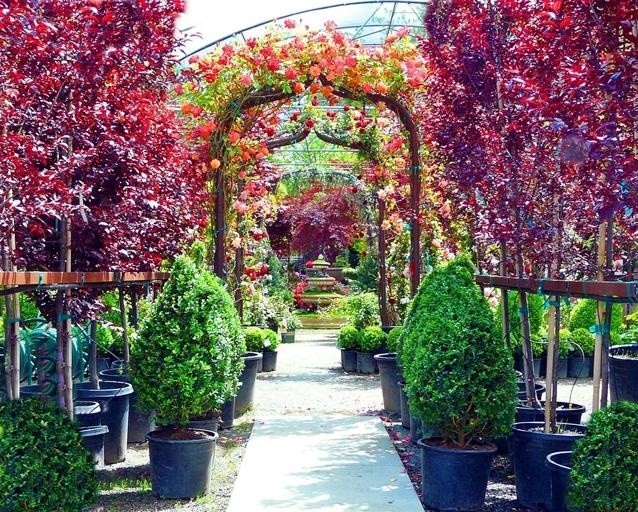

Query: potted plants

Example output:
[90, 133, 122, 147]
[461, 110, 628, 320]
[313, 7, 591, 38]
[335, 326, 388, 373]
[373, 0, 638, 511]
[0, 0, 301, 465]
[129, 254, 247, 498]
